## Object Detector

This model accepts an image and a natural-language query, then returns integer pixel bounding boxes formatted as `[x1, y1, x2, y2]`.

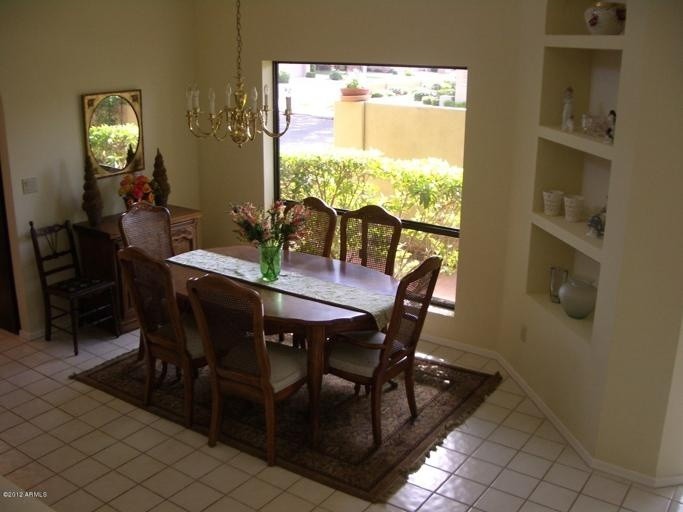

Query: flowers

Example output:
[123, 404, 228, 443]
[118, 175, 156, 209]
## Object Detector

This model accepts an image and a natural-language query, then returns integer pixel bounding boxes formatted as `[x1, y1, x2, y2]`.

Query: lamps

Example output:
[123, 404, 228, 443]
[184, 0, 293, 149]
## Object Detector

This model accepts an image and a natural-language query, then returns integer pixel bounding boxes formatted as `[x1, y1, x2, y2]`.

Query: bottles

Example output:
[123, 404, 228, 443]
[561, 84, 575, 132]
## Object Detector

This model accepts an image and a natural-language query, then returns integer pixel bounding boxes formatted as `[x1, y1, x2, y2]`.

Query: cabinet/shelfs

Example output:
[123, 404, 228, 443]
[73, 204, 205, 340]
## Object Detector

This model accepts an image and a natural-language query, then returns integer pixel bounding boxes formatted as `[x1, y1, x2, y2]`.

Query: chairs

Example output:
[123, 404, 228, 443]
[29, 219, 121, 355]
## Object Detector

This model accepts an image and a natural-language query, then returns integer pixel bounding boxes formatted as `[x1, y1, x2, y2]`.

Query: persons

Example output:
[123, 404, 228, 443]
[560, 84, 576, 133]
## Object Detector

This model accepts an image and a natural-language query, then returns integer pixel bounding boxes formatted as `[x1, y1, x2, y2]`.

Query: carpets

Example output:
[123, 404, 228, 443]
[69, 335, 501, 506]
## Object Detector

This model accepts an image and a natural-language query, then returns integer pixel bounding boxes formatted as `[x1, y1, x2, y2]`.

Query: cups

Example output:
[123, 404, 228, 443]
[548, 266, 569, 306]
[562, 193, 586, 224]
[581, 111, 608, 136]
[540, 189, 564, 217]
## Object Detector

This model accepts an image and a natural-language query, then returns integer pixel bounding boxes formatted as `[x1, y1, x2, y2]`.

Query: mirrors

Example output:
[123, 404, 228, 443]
[80, 87, 145, 181]
